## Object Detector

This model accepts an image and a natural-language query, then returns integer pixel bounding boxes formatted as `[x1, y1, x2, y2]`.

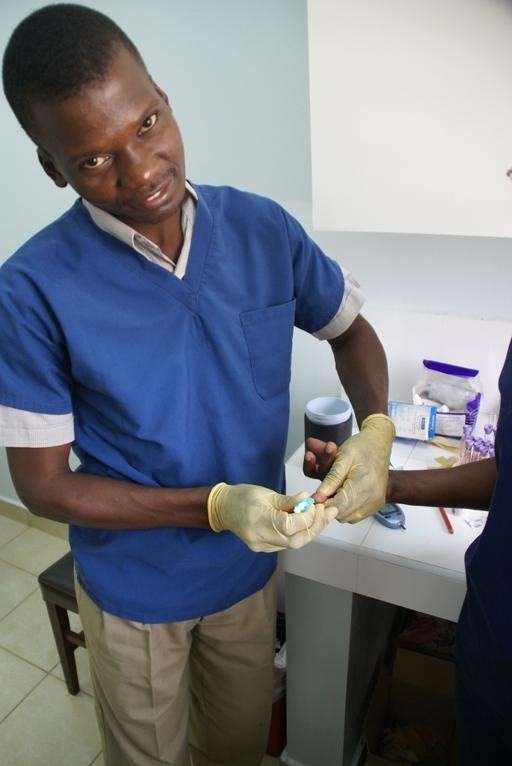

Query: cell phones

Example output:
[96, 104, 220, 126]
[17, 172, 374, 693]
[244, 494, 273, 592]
[372, 502, 406, 530]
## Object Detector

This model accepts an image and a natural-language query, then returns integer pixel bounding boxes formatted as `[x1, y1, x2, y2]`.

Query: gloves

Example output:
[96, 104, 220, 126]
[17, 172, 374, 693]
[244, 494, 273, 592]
[206, 481, 340, 554]
[310, 414, 398, 526]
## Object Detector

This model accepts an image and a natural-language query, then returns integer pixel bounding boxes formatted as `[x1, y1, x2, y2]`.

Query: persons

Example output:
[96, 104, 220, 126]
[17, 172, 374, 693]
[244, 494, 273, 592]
[1, 2, 396, 766]
[303, 337, 511, 765]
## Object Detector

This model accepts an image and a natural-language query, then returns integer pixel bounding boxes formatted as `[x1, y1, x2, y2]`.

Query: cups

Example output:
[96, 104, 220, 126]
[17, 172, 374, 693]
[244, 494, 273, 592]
[304, 397, 353, 445]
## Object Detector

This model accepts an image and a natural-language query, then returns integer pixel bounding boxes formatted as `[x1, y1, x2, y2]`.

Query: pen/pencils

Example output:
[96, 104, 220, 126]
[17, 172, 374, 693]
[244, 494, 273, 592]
[440, 507, 453, 534]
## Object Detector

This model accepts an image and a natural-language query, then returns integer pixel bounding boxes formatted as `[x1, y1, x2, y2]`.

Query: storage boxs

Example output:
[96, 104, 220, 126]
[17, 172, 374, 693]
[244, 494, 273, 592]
[359, 648, 461, 766]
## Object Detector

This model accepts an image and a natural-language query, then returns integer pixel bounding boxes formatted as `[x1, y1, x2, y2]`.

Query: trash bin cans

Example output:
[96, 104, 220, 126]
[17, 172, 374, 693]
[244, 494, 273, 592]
[266, 611, 286, 758]
